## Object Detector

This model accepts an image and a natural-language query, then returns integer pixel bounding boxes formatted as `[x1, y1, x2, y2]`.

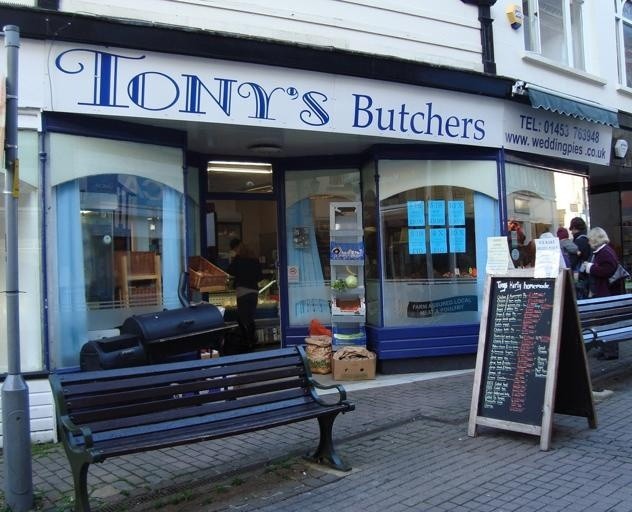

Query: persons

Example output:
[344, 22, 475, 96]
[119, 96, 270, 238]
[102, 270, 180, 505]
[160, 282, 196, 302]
[570, 216, 596, 297]
[554, 226, 582, 269]
[229, 237, 256, 268]
[222, 245, 260, 352]
[578, 224, 627, 361]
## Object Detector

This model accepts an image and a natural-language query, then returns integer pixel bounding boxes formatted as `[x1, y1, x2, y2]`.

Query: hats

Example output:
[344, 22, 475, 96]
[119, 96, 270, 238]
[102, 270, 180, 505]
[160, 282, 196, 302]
[570, 217, 587, 230]
[557, 227, 569, 239]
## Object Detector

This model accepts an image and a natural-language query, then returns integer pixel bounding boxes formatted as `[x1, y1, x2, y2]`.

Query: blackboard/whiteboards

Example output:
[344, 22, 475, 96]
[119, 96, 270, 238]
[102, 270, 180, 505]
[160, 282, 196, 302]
[471, 269, 565, 436]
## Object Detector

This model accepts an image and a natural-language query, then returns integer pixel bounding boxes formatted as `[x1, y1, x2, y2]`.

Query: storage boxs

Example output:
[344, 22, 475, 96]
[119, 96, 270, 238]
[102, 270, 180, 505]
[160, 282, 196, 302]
[332, 345, 378, 381]
[188, 255, 231, 293]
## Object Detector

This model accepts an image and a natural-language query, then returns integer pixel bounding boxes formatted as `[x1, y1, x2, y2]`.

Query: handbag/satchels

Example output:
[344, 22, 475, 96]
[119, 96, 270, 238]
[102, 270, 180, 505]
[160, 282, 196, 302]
[609, 263, 628, 287]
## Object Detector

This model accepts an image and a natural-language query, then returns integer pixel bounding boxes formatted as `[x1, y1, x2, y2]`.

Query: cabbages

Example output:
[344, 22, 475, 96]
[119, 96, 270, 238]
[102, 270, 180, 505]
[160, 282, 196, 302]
[346, 275, 358, 289]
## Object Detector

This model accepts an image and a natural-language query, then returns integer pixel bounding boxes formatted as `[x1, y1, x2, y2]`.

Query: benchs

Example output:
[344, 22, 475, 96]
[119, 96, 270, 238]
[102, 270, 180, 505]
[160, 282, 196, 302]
[575, 293, 631, 405]
[48, 345, 355, 512]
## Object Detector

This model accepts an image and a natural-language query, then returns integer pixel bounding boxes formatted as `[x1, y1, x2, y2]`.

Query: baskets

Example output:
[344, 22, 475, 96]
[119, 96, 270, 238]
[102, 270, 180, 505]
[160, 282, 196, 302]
[113, 251, 159, 308]
[185, 255, 230, 288]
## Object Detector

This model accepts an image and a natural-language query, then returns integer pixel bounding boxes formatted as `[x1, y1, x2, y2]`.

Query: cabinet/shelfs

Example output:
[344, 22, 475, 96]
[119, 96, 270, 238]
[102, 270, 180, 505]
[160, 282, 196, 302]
[112, 250, 162, 309]
[209, 297, 280, 351]
[330, 201, 367, 351]
